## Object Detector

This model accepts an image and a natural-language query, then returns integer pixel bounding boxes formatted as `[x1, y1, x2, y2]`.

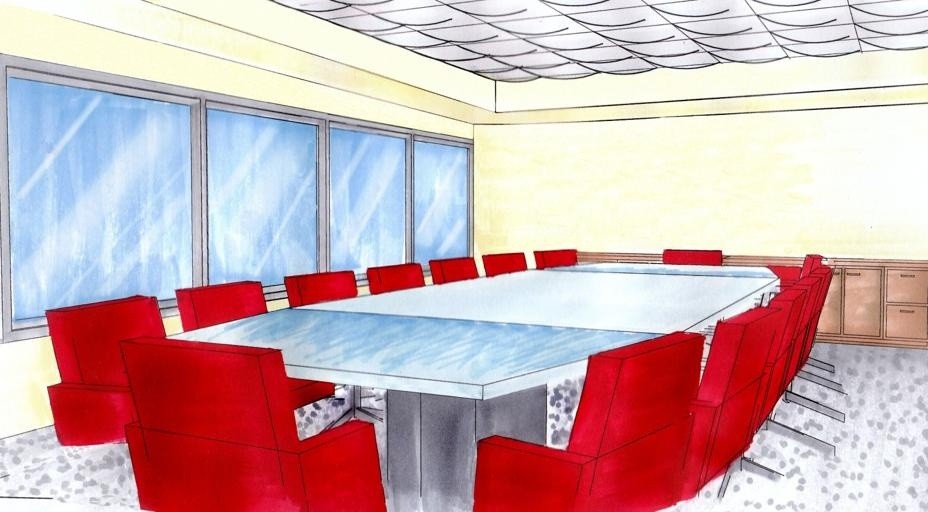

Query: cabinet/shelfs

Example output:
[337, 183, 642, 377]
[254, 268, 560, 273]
[815, 260, 928, 350]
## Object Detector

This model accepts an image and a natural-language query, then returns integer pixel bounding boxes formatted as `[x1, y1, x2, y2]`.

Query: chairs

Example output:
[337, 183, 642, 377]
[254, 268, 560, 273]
[43, 245, 848, 512]
